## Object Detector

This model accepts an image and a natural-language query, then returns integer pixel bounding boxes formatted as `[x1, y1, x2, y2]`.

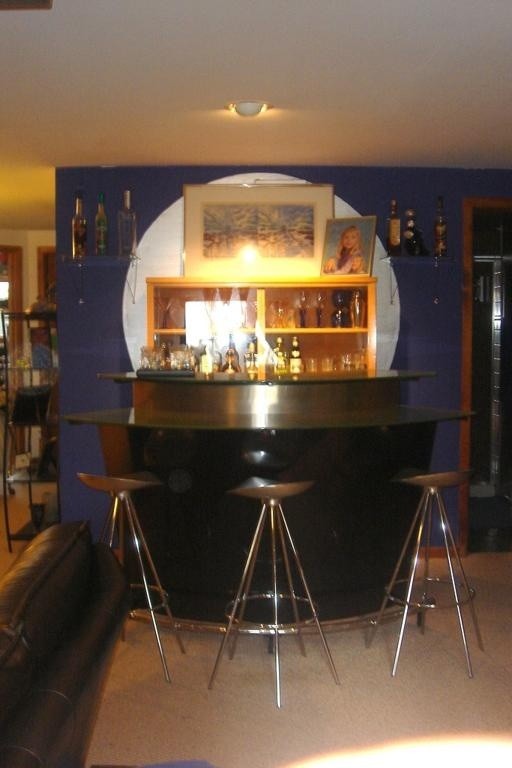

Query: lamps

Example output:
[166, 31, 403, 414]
[226, 101, 271, 119]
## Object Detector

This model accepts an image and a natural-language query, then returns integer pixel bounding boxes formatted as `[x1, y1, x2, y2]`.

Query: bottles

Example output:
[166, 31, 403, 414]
[385, 198, 403, 256]
[117, 190, 137, 256]
[403, 208, 423, 257]
[264, 336, 290, 379]
[94, 192, 109, 257]
[228, 333, 240, 365]
[289, 336, 303, 374]
[222, 350, 241, 374]
[200, 345, 213, 375]
[244, 341, 258, 368]
[71, 190, 89, 259]
[245, 351, 259, 374]
[433, 195, 448, 256]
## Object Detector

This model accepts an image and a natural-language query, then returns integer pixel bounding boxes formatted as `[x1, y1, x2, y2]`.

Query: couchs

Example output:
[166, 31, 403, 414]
[0, 515, 130, 767]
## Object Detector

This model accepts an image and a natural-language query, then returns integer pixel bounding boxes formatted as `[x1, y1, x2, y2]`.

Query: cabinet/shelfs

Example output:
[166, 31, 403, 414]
[145, 278, 377, 379]
[56, 256, 140, 274]
[381, 254, 458, 270]
[1, 311, 62, 554]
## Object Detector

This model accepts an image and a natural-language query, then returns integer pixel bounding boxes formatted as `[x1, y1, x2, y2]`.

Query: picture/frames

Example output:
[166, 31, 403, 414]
[182, 184, 336, 280]
[319, 215, 378, 276]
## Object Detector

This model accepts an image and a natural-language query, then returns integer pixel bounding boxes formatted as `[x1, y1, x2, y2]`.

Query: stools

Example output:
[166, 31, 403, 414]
[208, 481, 341, 708]
[366, 471, 486, 679]
[75, 469, 186, 683]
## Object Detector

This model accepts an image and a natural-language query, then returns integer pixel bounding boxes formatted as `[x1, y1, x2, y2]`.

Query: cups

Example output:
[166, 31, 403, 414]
[321, 358, 333, 372]
[355, 353, 366, 370]
[306, 358, 317, 373]
[340, 353, 351, 371]
